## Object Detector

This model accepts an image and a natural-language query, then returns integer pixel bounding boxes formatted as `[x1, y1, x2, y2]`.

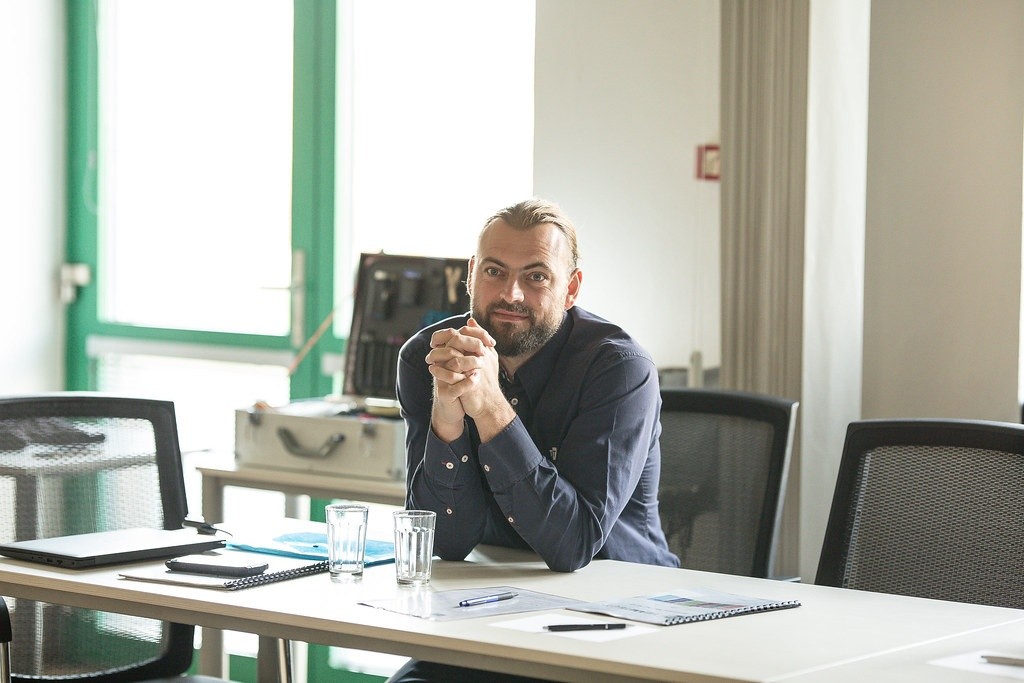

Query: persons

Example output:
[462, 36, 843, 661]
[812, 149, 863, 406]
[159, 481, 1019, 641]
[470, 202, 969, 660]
[384, 199, 683, 683]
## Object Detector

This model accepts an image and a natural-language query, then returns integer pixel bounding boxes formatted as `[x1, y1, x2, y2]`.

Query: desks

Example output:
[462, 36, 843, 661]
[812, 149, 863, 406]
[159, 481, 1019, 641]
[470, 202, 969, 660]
[198, 458, 407, 681]
[0, 425, 216, 671]
[0, 517, 1024, 683]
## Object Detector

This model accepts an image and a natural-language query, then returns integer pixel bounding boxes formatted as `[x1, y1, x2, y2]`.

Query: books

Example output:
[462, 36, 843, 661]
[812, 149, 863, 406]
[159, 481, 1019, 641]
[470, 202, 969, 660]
[566, 591, 801, 627]
[120, 547, 330, 590]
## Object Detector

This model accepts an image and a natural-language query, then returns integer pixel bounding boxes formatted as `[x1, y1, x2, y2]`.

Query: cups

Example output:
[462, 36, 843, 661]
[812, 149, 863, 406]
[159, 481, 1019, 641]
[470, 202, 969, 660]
[393, 510, 436, 584]
[324, 503, 368, 577]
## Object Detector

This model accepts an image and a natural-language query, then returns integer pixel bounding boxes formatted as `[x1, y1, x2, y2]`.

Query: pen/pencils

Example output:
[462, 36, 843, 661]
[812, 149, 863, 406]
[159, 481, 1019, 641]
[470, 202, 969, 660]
[455, 588, 523, 609]
[543, 618, 629, 634]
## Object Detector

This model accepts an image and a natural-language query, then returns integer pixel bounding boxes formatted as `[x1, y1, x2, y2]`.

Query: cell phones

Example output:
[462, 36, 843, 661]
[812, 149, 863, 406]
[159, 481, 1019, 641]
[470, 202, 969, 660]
[166, 554, 269, 577]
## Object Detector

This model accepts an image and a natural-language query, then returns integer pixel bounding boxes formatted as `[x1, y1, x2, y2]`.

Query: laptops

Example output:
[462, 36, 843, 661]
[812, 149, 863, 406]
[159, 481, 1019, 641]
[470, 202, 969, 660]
[0, 527, 227, 569]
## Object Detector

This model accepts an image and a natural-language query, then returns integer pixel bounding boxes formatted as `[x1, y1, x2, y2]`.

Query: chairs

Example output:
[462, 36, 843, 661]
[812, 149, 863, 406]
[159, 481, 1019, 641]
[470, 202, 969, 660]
[813, 419, 1024, 610]
[0, 392, 241, 683]
[657, 385, 801, 584]
